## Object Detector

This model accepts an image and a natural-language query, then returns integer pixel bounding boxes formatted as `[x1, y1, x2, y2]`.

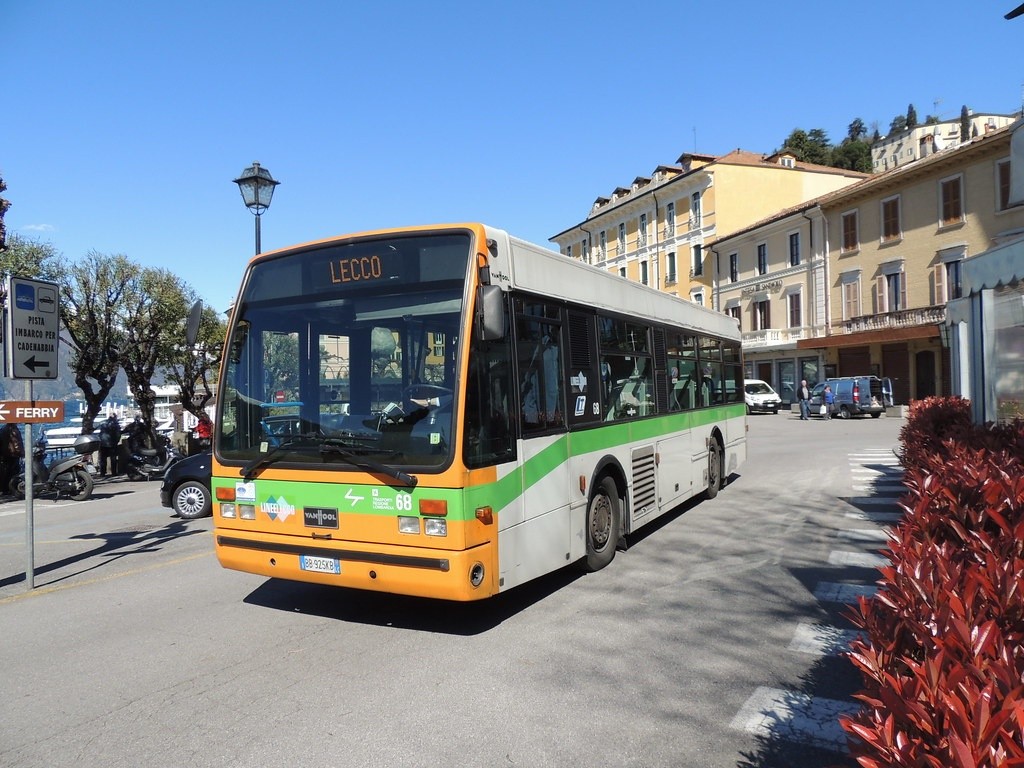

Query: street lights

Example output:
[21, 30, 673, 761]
[230, 161, 282, 256]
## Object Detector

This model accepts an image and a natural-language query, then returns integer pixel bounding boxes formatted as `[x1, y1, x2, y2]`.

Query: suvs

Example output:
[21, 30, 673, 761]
[160, 413, 346, 520]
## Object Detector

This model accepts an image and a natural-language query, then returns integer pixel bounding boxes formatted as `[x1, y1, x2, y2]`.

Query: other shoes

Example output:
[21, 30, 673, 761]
[800, 415, 803, 420]
[805, 418, 809, 420]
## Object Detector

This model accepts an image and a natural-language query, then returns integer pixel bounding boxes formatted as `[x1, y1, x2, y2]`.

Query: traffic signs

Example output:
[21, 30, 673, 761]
[6, 274, 59, 380]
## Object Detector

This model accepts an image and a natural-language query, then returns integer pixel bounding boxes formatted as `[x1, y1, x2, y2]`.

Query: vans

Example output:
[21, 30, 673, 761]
[717, 379, 782, 415]
[807, 376, 894, 420]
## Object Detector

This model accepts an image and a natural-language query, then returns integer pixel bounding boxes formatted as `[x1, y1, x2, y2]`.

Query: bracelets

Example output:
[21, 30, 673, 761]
[428, 398, 431, 406]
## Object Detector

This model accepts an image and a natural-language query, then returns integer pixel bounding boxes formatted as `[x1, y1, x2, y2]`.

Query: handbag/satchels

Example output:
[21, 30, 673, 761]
[820, 405, 826, 415]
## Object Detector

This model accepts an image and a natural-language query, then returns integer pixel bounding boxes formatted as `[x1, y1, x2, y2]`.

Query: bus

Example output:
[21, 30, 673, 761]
[187, 222, 748, 602]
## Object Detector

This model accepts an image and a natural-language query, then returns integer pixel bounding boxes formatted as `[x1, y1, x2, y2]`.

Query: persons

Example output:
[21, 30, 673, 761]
[796, 380, 813, 420]
[410, 394, 453, 408]
[194, 412, 212, 446]
[820, 384, 835, 420]
[0, 423, 24, 497]
[92, 413, 159, 479]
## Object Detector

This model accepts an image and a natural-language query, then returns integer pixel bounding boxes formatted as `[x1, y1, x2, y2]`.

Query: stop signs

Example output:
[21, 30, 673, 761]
[276, 390, 285, 402]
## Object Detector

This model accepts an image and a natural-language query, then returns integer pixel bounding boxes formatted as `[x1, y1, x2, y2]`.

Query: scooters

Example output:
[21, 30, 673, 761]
[9, 433, 102, 502]
[121, 433, 185, 482]
